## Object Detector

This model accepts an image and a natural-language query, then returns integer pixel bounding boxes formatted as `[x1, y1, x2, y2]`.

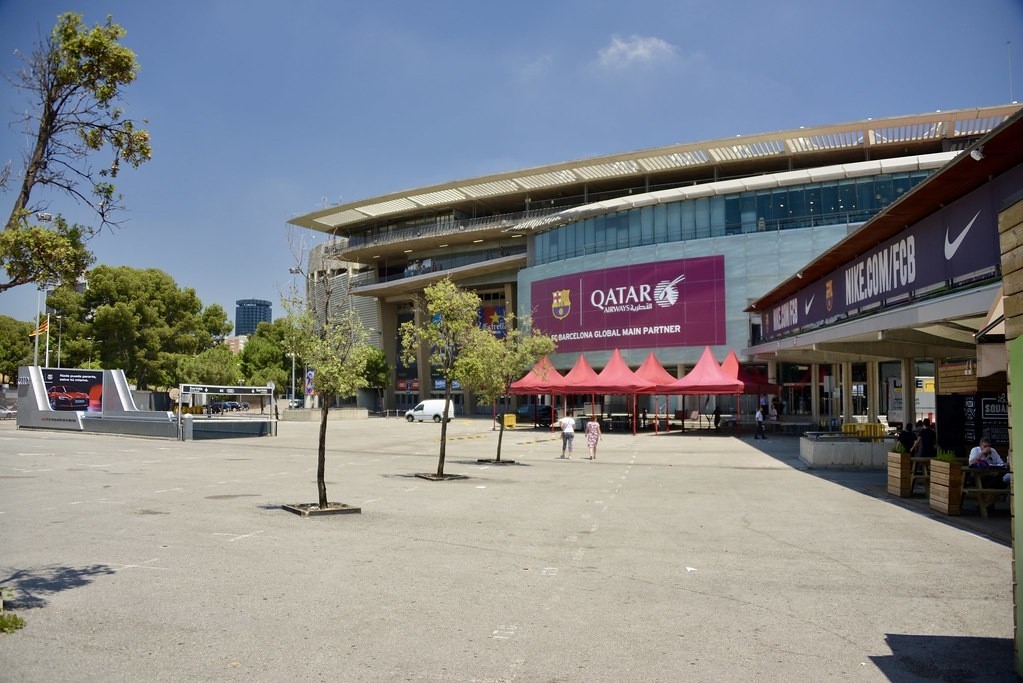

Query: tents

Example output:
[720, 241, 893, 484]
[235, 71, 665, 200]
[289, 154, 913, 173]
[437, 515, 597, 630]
[492, 354, 574, 431]
[550, 345, 781, 439]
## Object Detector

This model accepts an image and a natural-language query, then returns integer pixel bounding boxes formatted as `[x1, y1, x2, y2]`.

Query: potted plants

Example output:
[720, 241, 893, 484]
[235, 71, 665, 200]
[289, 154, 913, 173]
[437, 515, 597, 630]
[888, 442, 911, 498]
[929, 446, 960, 514]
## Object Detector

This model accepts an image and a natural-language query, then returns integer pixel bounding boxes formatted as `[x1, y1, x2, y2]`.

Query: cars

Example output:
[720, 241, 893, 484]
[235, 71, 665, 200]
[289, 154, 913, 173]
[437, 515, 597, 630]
[47, 385, 90, 410]
[0, 406, 17, 419]
[289, 399, 304, 407]
[211, 400, 250, 412]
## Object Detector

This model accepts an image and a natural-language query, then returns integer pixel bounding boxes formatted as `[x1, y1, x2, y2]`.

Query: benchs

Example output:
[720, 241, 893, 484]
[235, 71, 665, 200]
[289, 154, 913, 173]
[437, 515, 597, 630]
[913, 475, 930, 478]
[963, 489, 1011, 496]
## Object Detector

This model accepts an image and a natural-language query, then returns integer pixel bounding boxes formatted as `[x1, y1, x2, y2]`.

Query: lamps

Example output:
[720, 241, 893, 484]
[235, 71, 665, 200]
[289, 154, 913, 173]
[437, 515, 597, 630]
[797, 272, 803, 279]
[970, 147, 984, 160]
[752, 303, 757, 309]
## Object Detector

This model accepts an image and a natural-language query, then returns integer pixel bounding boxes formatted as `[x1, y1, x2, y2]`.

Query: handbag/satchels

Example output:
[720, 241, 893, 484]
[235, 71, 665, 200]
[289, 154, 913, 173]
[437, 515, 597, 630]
[559, 433, 563, 439]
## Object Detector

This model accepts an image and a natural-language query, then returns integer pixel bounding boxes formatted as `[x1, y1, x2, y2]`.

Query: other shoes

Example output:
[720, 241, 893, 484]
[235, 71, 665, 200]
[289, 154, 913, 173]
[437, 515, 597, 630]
[561, 455, 565, 458]
[568, 457, 571, 459]
[763, 437, 768, 439]
[590, 456, 592, 460]
[594, 457, 596, 459]
[755, 438, 759, 439]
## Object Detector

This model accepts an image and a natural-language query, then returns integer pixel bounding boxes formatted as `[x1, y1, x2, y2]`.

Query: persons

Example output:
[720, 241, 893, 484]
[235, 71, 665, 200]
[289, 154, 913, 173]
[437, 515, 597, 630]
[968, 438, 1007, 516]
[897, 423, 917, 457]
[712, 407, 723, 433]
[584, 416, 603, 459]
[770, 393, 779, 409]
[754, 407, 768, 440]
[915, 420, 923, 432]
[910, 419, 936, 457]
[760, 395, 768, 420]
[768, 405, 778, 432]
[560, 410, 575, 459]
[642, 408, 647, 430]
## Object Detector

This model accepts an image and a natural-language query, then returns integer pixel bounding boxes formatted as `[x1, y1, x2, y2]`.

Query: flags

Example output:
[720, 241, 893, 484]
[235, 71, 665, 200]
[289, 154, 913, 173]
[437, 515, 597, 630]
[28, 319, 48, 336]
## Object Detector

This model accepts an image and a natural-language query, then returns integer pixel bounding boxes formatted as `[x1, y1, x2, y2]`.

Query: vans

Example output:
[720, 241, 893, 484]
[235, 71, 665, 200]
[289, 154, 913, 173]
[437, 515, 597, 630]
[403, 399, 455, 423]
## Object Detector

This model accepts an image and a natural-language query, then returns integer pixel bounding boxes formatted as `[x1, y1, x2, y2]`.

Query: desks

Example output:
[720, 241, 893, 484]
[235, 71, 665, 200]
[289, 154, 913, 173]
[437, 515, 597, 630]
[910, 457, 930, 501]
[961, 466, 1010, 519]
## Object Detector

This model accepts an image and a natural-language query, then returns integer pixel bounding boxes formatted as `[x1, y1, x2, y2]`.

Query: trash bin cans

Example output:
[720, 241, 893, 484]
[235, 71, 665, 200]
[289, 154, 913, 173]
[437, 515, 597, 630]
[503, 413, 516, 429]
